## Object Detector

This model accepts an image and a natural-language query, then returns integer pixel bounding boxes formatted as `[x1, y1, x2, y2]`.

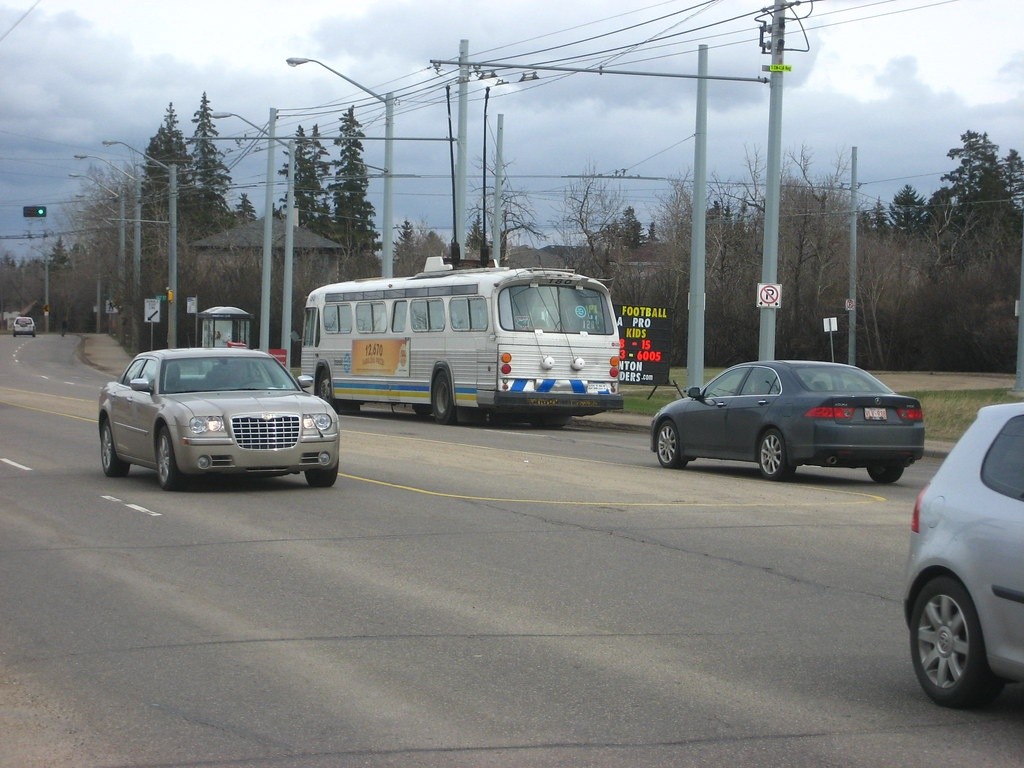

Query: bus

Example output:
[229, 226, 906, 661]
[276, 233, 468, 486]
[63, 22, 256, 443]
[289, 253, 624, 427]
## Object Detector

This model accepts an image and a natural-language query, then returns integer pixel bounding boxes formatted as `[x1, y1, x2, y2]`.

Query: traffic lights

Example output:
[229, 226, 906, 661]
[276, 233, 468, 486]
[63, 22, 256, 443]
[23, 206, 47, 218]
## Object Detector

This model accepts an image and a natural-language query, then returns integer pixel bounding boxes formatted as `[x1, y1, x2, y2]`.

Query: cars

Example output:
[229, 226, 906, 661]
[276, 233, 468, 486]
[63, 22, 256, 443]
[650, 359, 926, 483]
[13, 316, 37, 338]
[901, 399, 1024, 712]
[97, 346, 340, 490]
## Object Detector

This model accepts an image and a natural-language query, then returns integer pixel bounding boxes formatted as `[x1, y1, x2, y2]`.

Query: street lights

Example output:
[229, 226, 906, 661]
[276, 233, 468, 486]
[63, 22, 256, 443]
[74, 154, 143, 356]
[209, 109, 295, 375]
[103, 138, 178, 349]
[286, 56, 396, 280]
[68, 174, 126, 344]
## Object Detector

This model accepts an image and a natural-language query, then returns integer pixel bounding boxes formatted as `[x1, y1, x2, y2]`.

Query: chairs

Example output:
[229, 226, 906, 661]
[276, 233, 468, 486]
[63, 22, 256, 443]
[813, 381, 829, 391]
